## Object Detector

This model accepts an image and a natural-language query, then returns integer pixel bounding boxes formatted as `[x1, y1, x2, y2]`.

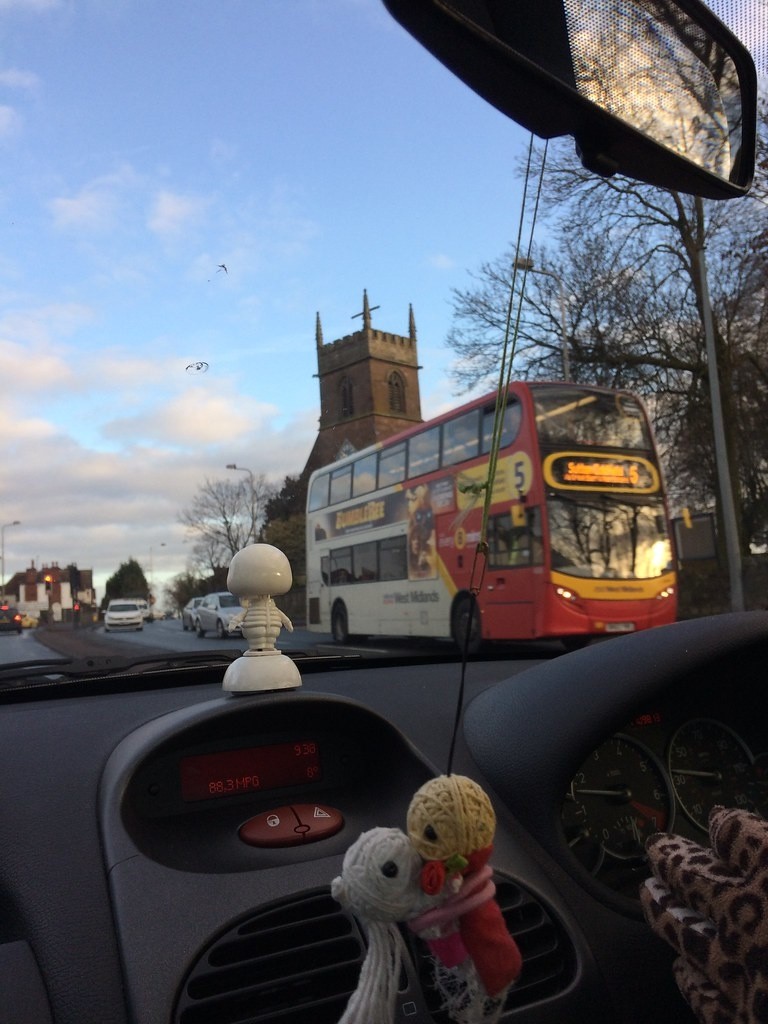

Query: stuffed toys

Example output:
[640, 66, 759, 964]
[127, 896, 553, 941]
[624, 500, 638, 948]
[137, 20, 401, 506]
[330, 775, 523, 1024]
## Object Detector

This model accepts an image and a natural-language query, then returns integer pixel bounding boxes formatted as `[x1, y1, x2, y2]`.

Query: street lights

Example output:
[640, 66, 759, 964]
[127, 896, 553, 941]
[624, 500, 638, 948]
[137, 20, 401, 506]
[0, 521, 20, 584]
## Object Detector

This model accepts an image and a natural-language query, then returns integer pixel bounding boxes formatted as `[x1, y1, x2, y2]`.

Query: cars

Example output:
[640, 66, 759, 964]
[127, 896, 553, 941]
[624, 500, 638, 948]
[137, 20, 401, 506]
[101, 598, 152, 631]
[195, 592, 247, 640]
[0, 605, 22, 634]
[182, 598, 202, 631]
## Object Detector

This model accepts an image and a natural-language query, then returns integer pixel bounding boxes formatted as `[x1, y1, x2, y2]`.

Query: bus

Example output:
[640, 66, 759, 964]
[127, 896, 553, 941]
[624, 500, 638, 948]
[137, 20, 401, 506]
[306, 383, 679, 657]
